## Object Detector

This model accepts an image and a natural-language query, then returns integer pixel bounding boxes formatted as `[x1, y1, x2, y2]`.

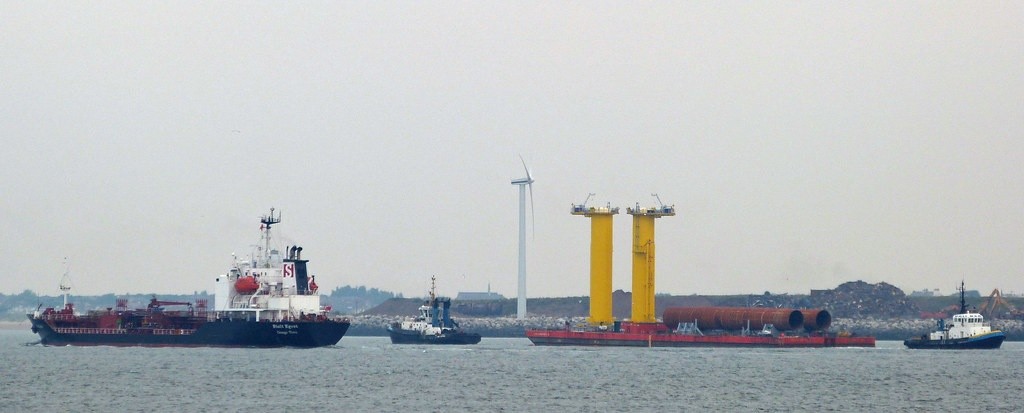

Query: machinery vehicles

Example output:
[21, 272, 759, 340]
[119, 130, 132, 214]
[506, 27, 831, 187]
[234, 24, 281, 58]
[978, 288, 1024, 319]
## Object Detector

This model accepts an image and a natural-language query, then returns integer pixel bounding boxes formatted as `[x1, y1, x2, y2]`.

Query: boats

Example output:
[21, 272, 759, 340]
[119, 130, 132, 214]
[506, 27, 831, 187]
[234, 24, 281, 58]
[526, 193, 875, 347]
[26, 207, 351, 348]
[386, 275, 482, 345]
[903, 271, 1005, 350]
